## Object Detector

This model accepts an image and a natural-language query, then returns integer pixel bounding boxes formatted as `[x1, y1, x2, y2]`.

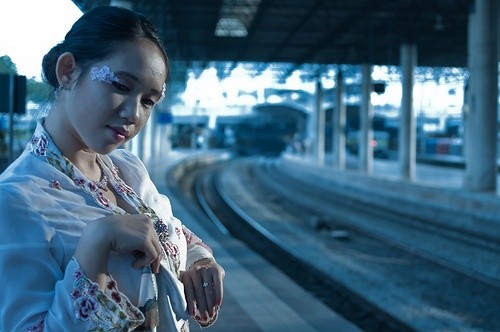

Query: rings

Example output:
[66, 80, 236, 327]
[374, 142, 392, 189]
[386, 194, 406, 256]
[203, 282, 215, 288]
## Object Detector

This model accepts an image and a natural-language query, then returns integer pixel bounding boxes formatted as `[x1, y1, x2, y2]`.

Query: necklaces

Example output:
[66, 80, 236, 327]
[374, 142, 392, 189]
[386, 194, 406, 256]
[88, 167, 109, 191]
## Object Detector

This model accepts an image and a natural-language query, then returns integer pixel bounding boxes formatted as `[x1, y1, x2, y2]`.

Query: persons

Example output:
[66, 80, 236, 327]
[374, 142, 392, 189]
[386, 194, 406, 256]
[0, 5, 226, 332]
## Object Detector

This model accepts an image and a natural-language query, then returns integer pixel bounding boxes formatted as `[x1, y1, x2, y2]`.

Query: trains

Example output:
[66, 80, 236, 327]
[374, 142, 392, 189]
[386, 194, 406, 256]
[387, 117, 500, 169]
[346, 113, 389, 157]
